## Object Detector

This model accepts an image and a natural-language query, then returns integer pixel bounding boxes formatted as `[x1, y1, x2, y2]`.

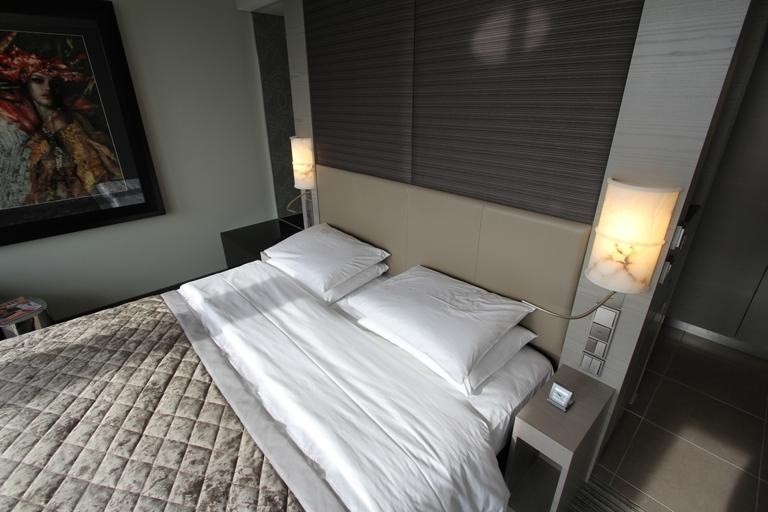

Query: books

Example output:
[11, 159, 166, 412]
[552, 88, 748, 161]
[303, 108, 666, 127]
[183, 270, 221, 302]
[0, 295, 42, 327]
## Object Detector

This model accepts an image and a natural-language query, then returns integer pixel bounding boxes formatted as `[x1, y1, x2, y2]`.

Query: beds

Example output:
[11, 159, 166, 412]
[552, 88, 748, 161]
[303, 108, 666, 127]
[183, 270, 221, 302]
[0, 223, 555, 512]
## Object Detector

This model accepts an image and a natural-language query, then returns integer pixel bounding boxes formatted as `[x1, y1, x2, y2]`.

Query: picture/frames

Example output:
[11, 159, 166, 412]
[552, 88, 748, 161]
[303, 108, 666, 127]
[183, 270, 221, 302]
[0, 0, 169, 247]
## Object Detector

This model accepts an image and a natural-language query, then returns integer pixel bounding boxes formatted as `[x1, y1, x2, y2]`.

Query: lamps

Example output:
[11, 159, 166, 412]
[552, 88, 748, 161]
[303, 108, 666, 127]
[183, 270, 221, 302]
[285, 133, 317, 215]
[521, 174, 682, 322]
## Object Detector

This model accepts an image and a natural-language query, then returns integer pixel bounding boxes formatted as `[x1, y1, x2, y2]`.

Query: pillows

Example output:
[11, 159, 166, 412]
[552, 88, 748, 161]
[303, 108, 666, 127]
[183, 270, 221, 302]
[265, 257, 390, 305]
[346, 264, 537, 387]
[263, 222, 390, 293]
[356, 317, 540, 396]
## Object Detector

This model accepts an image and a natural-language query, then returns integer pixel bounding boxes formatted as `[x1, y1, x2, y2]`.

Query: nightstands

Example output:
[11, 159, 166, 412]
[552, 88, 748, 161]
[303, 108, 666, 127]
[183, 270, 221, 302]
[215, 210, 314, 270]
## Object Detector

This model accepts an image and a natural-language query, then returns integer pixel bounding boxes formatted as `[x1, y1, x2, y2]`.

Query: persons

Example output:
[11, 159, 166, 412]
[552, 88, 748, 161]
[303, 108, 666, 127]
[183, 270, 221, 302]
[1, 30, 127, 208]
[13, 296, 41, 315]
[1, 308, 23, 321]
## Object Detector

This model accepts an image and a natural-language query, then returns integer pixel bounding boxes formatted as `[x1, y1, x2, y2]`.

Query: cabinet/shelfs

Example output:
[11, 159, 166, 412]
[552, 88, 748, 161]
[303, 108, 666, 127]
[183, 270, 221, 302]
[664, 39, 767, 362]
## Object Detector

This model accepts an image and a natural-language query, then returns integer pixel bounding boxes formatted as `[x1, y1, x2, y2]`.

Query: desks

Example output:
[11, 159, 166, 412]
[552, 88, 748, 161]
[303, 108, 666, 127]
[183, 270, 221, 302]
[0, 296, 49, 338]
[502, 364, 616, 511]
